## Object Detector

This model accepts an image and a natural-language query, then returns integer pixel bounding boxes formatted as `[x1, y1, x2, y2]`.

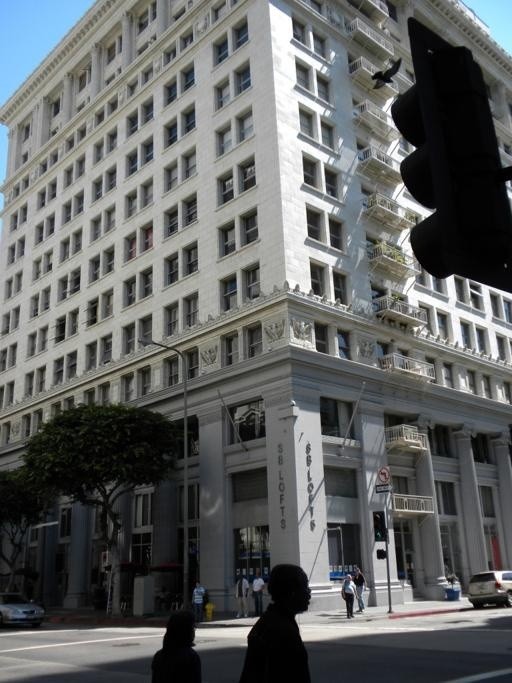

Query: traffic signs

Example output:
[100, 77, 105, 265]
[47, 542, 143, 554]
[376, 482, 394, 494]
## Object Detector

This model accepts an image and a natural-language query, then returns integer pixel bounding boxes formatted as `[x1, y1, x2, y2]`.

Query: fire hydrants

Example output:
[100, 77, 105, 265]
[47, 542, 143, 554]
[204, 603, 217, 621]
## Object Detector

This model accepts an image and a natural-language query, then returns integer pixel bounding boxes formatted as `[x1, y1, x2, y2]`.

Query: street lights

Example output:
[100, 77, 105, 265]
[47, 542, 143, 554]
[136, 336, 193, 613]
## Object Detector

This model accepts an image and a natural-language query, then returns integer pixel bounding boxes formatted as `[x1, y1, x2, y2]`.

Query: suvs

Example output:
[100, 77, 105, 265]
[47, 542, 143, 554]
[467, 569, 512, 610]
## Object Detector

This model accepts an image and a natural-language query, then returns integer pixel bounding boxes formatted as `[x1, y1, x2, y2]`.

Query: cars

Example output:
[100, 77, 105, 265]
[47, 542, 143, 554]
[1, 592, 46, 628]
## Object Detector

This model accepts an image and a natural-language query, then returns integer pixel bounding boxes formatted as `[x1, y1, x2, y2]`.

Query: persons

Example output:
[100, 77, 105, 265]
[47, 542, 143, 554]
[152, 609, 202, 682]
[238, 562, 313, 682]
[191, 580, 206, 622]
[234, 570, 249, 618]
[353, 566, 367, 613]
[340, 573, 356, 619]
[251, 571, 265, 617]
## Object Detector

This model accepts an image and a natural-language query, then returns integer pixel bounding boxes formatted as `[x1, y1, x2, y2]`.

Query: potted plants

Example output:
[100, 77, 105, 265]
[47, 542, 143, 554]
[372, 239, 384, 257]
[444, 574, 461, 601]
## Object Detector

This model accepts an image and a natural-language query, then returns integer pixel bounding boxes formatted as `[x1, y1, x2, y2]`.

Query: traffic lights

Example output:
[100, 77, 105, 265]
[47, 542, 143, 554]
[387, 15, 511, 294]
[372, 510, 386, 541]
